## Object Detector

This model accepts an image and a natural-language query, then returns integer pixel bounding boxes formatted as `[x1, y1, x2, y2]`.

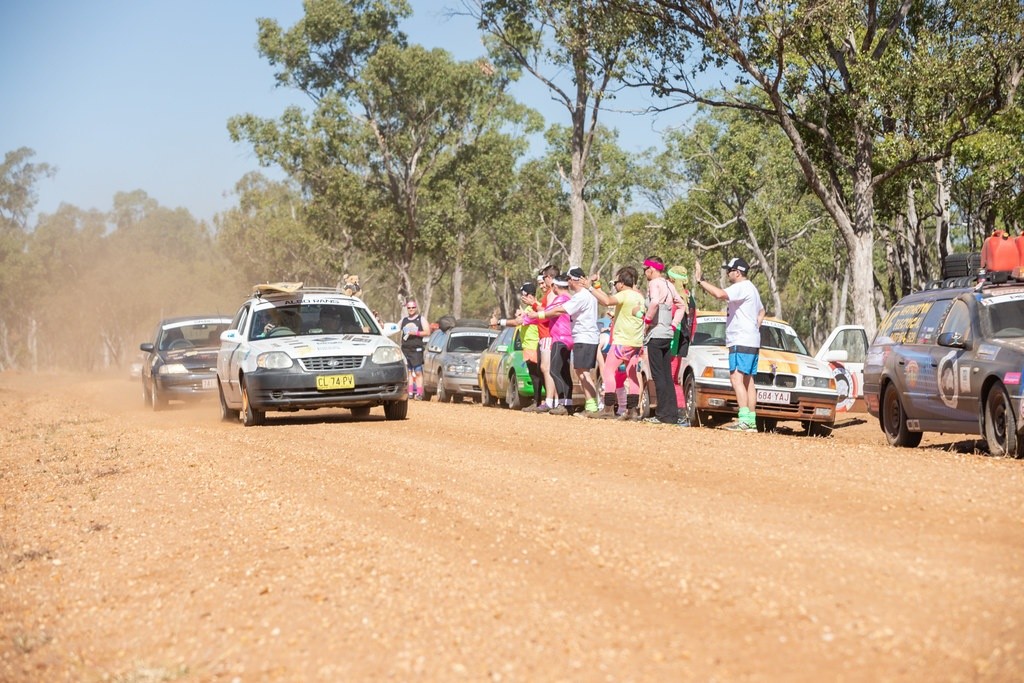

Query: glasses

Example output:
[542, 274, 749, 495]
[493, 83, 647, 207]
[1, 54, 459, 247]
[613, 281, 621, 286]
[407, 307, 416, 309]
[728, 268, 736, 271]
[643, 266, 650, 274]
[542, 275, 548, 281]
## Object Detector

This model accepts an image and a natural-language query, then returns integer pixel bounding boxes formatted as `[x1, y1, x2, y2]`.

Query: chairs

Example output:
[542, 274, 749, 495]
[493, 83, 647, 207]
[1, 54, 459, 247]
[691, 332, 712, 346]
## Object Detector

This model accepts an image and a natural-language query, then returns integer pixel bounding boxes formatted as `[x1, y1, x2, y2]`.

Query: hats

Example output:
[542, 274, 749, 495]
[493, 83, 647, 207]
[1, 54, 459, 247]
[666, 265, 689, 284]
[520, 283, 536, 294]
[721, 258, 750, 273]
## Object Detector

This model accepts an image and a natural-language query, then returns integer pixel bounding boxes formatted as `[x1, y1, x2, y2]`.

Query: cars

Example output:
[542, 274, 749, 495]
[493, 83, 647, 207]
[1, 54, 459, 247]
[475, 327, 602, 411]
[139, 316, 235, 412]
[216, 286, 410, 427]
[421, 326, 503, 404]
[640, 309, 871, 437]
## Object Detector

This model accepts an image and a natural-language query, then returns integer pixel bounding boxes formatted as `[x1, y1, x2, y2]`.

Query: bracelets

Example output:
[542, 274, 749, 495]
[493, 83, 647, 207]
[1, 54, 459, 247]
[497, 320, 500, 325]
[588, 286, 593, 292]
[532, 303, 538, 310]
[698, 278, 705, 285]
[539, 311, 545, 319]
[593, 282, 600, 289]
[417, 331, 420, 336]
[501, 319, 507, 327]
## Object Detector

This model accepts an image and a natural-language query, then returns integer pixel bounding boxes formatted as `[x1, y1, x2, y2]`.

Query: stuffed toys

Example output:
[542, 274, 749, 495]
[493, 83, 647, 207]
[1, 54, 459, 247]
[345, 275, 363, 298]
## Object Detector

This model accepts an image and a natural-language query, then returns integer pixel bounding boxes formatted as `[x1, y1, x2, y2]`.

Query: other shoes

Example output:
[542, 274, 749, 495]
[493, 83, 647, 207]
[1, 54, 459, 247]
[416, 394, 422, 401]
[535, 403, 553, 412]
[522, 404, 536, 412]
[590, 405, 614, 418]
[617, 407, 637, 421]
[408, 393, 414, 398]
[549, 405, 568, 415]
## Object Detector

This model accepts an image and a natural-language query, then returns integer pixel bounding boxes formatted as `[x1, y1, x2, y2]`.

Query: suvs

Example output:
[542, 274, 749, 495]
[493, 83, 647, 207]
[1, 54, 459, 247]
[861, 252, 1024, 459]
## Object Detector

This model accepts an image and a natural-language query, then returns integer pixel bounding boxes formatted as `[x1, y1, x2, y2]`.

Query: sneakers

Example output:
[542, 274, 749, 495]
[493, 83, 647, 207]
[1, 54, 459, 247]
[643, 416, 664, 424]
[674, 415, 689, 427]
[717, 421, 758, 433]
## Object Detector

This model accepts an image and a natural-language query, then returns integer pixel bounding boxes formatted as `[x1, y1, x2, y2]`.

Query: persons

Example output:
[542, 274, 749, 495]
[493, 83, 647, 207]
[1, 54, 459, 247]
[616, 266, 645, 299]
[489, 267, 560, 413]
[397, 302, 431, 401]
[695, 258, 766, 432]
[328, 314, 341, 330]
[667, 265, 698, 425]
[527, 267, 599, 419]
[579, 271, 646, 422]
[542, 265, 559, 309]
[642, 256, 687, 424]
[263, 317, 282, 334]
[168, 330, 179, 342]
